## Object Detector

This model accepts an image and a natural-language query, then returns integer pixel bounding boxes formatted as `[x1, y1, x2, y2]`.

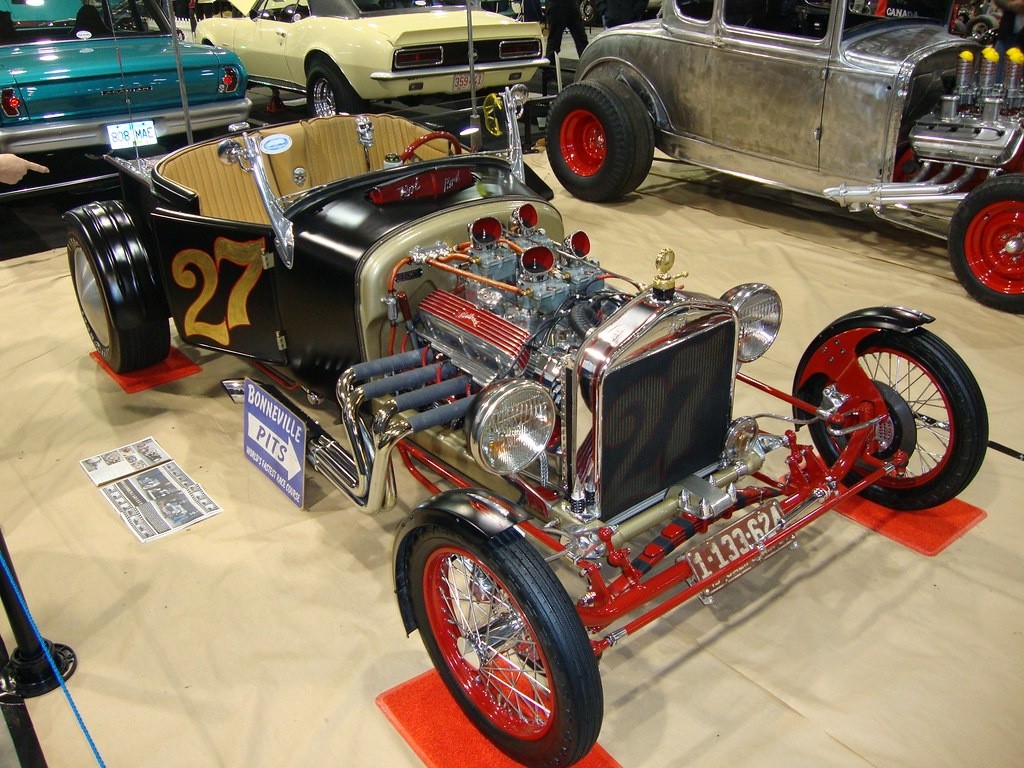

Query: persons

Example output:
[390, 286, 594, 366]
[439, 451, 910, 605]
[545, 0, 589, 66]
[604, 0, 649, 28]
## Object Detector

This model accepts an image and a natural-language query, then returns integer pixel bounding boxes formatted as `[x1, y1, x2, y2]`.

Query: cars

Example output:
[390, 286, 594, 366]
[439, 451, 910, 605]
[186, 0, 550, 121]
[544, 0, 1024, 314]
[0, 0, 252, 200]
[59, 82, 989, 768]
